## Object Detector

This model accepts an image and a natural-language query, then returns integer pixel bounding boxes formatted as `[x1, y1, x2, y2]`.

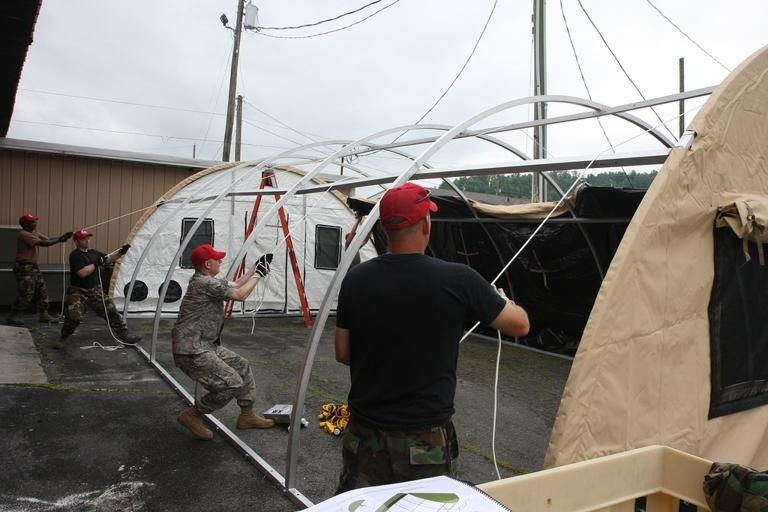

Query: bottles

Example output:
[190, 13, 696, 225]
[73, 230, 93, 241]
[19, 215, 38, 225]
[190, 244, 226, 266]
[379, 183, 438, 229]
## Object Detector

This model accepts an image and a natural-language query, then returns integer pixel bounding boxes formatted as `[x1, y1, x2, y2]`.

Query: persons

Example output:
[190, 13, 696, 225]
[16, 213, 73, 325]
[62, 230, 142, 347]
[336, 183, 529, 497]
[171, 244, 276, 439]
[345, 215, 371, 270]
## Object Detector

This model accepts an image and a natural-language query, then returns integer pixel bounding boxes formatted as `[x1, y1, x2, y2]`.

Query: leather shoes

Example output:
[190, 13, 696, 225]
[255, 262, 270, 277]
[492, 284, 510, 304]
[119, 244, 131, 254]
[256, 253, 273, 265]
[65, 232, 73, 239]
[59, 235, 69, 242]
[94, 254, 108, 268]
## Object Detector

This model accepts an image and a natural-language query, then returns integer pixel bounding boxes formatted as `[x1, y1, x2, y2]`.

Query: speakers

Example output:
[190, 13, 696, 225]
[39, 310, 58, 324]
[238, 405, 274, 430]
[115, 329, 142, 345]
[177, 406, 213, 439]
[6, 312, 24, 326]
[53, 332, 73, 349]
